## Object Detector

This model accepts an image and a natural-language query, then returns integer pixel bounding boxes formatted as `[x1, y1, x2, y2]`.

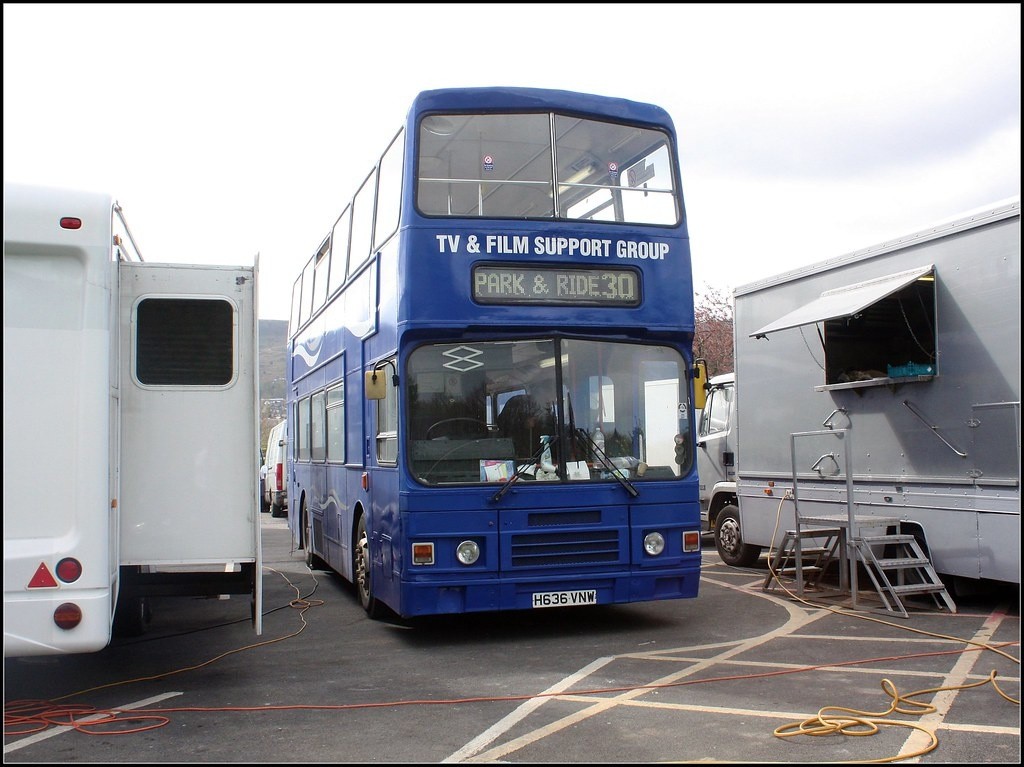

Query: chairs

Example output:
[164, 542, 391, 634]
[497, 395, 556, 458]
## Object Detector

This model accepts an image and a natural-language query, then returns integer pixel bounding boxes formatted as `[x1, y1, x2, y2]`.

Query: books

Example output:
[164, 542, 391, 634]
[480, 460, 514, 483]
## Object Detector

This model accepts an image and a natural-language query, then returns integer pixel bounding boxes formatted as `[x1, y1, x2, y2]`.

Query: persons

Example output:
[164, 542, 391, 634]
[497, 378, 557, 467]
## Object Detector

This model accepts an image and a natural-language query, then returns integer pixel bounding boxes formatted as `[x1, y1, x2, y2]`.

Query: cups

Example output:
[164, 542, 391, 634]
[637, 462, 648, 477]
[542, 462, 556, 473]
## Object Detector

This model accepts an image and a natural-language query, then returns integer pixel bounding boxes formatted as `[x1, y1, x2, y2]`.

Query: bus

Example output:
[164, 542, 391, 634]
[288, 85, 712, 621]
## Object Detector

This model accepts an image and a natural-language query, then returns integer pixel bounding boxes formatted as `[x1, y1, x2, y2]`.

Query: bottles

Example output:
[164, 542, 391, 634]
[600, 469, 630, 480]
[592, 428, 605, 469]
[605, 456, 641, 470]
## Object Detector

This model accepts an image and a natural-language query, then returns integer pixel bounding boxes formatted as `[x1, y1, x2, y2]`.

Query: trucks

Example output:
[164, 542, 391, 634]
[4, 177, 263, 654]
[692, 200, 1021, 586]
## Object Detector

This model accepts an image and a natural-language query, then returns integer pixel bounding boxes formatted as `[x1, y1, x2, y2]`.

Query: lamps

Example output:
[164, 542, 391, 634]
[607, 130, 643, 154]
[548, 164, 598, 200]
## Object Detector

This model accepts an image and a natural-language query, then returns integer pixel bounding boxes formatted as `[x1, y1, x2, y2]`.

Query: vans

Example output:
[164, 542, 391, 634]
[259, 419, 288, 517]
